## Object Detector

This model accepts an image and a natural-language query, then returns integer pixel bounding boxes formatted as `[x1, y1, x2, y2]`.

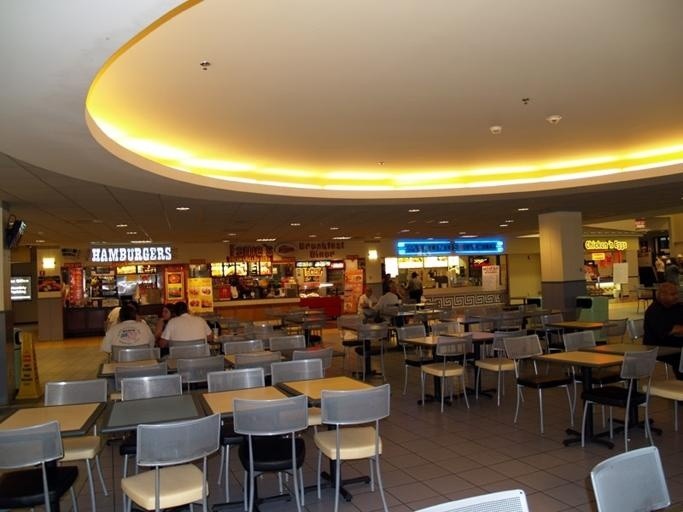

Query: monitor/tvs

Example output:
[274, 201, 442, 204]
[6, 219, 27, 250]
[655, 236, 667, 255]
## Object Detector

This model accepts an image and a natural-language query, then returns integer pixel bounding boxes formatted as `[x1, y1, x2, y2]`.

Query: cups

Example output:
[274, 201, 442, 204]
[213, 328, 219, 339]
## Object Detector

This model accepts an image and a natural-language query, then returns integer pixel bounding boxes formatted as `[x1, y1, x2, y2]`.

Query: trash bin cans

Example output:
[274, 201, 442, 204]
[575, 295, 608, 346]
[13, 328, 23, 390]
[525, 297, 543, 330]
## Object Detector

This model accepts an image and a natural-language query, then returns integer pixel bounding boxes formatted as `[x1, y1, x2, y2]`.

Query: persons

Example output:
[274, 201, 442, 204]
[159, 302, 213, 347]
[373, 286, 399, 320]
[405, 271, 424, 303]
[127, 302, 152, 333]
[389, 288, 402, 300]
[382, 274, 396, 296]
[642, 282, 683, 380]
[155, 303, 176, 341]
[106, 306, 122, 329]
[357, 287, 378, 321]
[101, 305, 155, 353]
